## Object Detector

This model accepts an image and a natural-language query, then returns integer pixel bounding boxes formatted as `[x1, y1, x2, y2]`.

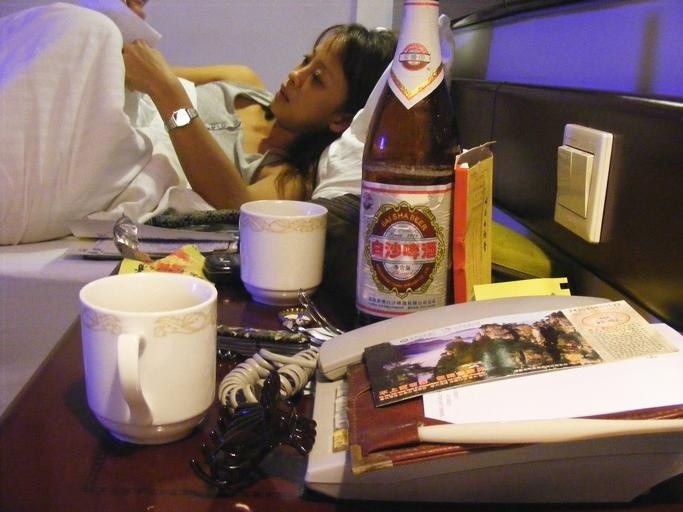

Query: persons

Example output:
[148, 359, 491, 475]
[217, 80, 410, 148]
[0, 0, 399, 247]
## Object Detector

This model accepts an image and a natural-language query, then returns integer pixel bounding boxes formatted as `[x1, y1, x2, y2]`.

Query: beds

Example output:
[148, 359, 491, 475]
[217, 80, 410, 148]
[1, 1, 682, 414]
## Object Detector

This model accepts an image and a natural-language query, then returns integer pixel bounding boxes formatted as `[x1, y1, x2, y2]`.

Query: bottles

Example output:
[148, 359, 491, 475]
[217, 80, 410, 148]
[350, 1, 464, 337]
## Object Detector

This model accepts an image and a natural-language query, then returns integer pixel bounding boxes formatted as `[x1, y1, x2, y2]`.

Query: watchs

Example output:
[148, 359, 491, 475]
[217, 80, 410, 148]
[162, 104, 197, 132]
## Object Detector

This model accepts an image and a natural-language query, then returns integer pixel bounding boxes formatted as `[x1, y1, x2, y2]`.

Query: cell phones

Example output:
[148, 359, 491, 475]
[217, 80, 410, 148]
[203, 248, 241, 282]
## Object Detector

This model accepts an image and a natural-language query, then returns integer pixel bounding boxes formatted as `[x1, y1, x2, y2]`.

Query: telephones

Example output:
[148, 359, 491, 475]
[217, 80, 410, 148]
[304, 295, 683, 503]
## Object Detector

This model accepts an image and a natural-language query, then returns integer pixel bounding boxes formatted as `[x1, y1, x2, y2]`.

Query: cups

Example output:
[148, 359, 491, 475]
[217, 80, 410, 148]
[75, 270, 222, 446]
[235, 197, 329, 311]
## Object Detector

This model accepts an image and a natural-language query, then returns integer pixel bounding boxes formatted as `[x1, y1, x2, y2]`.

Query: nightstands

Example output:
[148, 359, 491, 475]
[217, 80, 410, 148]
[0, 259, 681, 508]
